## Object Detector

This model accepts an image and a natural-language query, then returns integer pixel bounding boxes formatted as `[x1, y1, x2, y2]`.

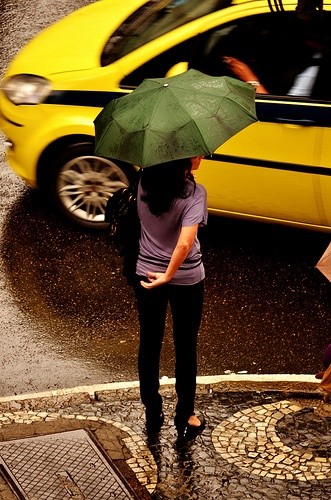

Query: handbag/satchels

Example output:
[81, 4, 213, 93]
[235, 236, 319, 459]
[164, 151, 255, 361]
[105, 167, 149, 288]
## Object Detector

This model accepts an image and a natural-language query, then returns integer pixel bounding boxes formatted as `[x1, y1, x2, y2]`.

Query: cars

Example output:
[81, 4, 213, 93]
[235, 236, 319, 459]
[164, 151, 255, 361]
[0, 0, 331, 236]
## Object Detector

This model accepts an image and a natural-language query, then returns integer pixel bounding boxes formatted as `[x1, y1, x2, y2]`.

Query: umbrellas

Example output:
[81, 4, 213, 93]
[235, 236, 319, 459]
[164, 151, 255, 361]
[93, 69, 259, 169]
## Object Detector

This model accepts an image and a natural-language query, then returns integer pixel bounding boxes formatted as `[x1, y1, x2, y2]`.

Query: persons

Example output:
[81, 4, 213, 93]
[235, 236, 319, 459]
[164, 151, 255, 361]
[134, 155, 207, 435]
[224, 24, 322, 98]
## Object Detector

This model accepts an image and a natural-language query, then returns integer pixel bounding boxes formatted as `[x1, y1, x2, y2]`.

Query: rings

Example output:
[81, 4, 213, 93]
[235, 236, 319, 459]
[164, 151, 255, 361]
[227, 59, 231, 64]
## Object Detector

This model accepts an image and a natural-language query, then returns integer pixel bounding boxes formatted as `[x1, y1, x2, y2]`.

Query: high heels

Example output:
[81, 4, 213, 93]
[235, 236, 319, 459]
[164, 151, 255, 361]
[174, 414, 205, 437]
[147, 411, 164, 433]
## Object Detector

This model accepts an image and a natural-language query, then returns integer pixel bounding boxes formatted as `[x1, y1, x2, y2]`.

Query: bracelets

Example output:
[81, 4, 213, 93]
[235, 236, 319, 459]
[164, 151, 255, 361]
[246, 81, 260, 88]
